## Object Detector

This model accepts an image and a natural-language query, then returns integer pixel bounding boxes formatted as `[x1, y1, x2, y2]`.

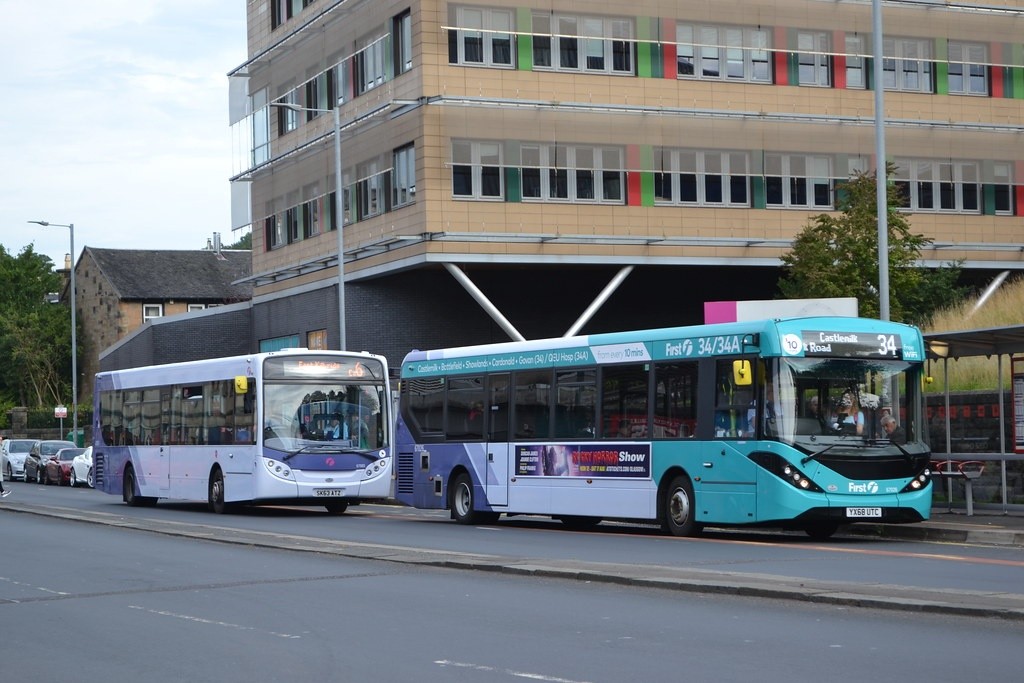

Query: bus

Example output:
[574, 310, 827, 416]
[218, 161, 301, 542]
[393, 315, 935, 538]
[94, 348, 396, 516]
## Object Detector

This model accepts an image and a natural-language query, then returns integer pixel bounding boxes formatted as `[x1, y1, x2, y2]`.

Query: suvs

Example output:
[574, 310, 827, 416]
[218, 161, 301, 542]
[23, 440, 79, 485]
[0, 439, 42, 482]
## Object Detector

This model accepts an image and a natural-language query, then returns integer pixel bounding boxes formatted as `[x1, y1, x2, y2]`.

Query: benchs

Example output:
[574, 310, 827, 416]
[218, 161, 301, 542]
[929, 461, 985, 516]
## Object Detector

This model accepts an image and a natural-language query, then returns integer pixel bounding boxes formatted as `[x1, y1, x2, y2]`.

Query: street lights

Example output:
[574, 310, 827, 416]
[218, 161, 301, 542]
[269, 102, 346, 351]
[28, 219, 83, 442]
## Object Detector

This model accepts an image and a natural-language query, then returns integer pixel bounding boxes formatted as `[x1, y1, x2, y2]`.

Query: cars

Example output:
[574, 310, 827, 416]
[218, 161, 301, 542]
[70, 446, 95, 489]
[43, 448, 87, 487]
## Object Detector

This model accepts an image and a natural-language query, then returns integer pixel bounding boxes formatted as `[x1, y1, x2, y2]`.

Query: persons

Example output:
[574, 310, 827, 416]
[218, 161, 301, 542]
[0, 437, 11, 497]
[617, 420, 634, 439]
[325, 411, 371, 448]
[266, 401, 304, 438]
[750, 377, 788, 436]
[808, 391, 912, 443]
